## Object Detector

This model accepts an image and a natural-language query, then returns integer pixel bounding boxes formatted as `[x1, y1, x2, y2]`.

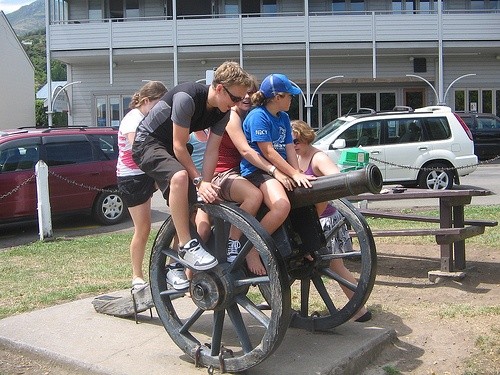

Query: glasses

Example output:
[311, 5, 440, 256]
[223, 85, 245, 103]
[294, 138, 301, 145]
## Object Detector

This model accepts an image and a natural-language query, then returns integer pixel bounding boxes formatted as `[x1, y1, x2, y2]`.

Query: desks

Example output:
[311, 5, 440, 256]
[347, 191, 493, 274]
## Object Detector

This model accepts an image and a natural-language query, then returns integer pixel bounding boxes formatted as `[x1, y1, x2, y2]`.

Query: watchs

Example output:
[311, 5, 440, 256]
[193, 177, 203, 185]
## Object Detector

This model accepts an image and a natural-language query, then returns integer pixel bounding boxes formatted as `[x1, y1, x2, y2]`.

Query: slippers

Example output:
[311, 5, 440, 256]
[129, 280, 149, 292]
[355, 312, 372, 321]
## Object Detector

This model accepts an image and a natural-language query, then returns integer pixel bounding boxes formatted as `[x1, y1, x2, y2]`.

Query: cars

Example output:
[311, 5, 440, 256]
[454, 110, 500, 160]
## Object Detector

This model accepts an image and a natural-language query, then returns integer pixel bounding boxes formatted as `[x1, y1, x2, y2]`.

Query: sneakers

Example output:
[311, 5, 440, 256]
[227, 238, 241, 262]
[165, 262, 191, 290]
[177, 239, 218, 270]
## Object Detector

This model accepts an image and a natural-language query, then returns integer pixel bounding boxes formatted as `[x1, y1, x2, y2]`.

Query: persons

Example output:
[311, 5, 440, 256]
[211, 77, 258, 263]
[239, 73, 318, 276]
[132, 60, 251, 290]
[115, 81, 169, 290]
[183, 129, 210, 281]
[288, 119, 372, 322]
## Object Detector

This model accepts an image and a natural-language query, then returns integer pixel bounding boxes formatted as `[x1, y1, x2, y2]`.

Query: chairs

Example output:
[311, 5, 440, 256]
[398, 122, 420, 143]
[2, 148, 37, 172]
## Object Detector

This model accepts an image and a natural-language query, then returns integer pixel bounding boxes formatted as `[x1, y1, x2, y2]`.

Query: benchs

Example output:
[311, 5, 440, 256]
[358, 208, 498, 228]
[346, 229, 475, 238]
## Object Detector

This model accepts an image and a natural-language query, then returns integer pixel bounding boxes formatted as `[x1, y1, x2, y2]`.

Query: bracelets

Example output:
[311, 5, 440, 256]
[268, 166, 276, 177]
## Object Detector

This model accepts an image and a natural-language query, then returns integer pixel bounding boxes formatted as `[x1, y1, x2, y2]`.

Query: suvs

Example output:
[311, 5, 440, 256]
[311, 104, 478, 190]
[0, 124, 126, 228]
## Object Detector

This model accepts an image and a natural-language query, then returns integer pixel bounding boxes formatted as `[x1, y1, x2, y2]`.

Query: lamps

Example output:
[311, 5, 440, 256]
[201, 60, 207, 65]
[112, 64, 117, 68]
[408, 55, 415, 63]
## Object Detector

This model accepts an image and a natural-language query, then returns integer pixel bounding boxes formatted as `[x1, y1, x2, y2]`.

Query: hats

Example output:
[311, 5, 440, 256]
[260, 74, 300, 99]
